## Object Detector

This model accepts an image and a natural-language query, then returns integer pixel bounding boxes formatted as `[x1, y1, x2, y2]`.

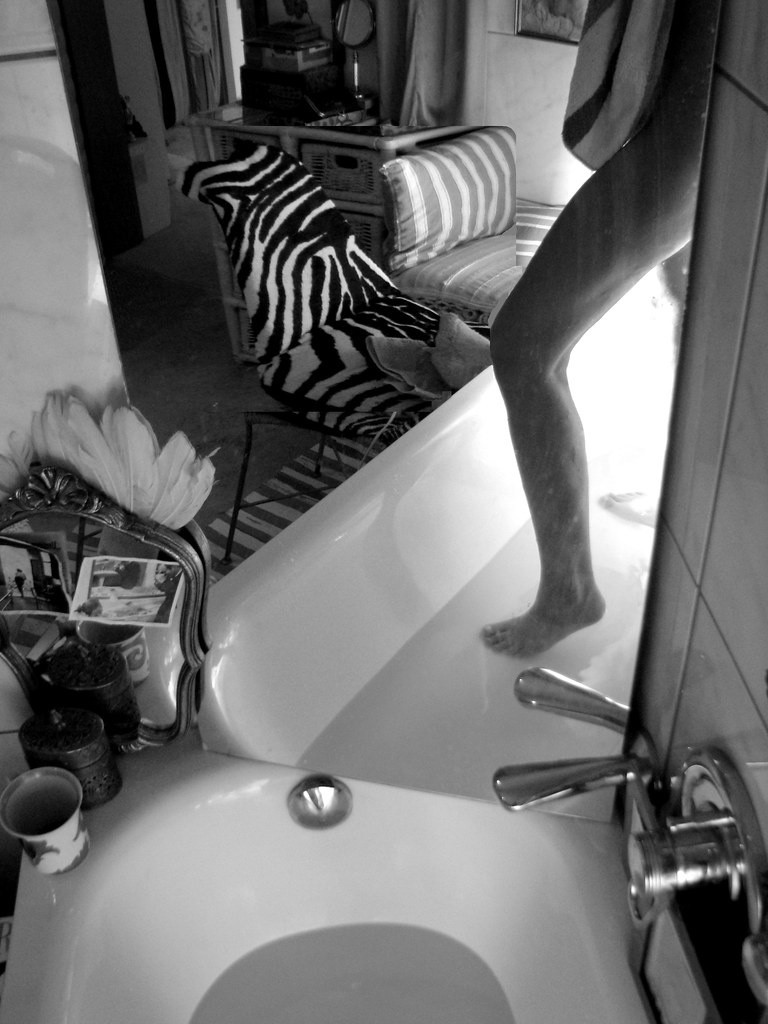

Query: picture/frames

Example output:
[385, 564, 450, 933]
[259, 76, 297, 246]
[514, 0, 589, 45]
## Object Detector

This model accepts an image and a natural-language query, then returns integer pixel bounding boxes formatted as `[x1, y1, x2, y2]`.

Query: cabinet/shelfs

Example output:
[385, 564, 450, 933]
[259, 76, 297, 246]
[187, 101, 465, 365]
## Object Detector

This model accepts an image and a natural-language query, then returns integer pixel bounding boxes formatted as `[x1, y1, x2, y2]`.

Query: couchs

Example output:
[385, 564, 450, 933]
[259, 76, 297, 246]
[390, 197, 566, 324]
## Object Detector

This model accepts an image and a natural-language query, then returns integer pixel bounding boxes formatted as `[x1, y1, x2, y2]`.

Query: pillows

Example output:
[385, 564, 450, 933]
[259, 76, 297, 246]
[379, 125, 517, 272]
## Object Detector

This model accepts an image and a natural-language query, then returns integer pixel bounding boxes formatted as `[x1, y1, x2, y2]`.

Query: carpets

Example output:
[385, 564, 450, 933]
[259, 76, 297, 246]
[207, 434, 378, 585]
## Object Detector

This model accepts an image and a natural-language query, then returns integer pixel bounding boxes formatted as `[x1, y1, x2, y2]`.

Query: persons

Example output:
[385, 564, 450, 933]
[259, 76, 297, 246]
[154, 562, 182, 623]
[78, 599, 103, 617]
[114, 560, 140, 589]
[481, 0, 718, 655]
[14, 569, 26, 598]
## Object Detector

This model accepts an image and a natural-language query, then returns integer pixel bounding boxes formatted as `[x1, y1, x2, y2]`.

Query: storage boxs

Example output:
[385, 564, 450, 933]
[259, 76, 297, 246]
[240, 63, 342, 126]
[244, 40, 331, 71]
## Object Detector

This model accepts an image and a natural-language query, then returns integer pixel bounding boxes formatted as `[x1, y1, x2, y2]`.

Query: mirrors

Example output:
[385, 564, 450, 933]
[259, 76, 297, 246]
[0, 0, 723, 818]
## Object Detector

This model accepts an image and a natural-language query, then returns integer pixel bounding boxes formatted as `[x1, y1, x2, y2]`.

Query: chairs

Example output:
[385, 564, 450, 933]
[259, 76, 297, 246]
[181, 144, 491, 577]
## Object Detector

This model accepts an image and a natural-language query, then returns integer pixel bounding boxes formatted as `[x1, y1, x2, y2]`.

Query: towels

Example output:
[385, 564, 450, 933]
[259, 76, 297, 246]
[560, 0, 675, 173]
[365, 308, 496, 401]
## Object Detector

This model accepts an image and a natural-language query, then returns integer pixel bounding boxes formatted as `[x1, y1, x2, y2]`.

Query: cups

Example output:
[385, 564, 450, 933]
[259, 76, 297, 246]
[46, 643, 141, 742]
[76, 620, 150, 687]
[0, 766, 91, 876]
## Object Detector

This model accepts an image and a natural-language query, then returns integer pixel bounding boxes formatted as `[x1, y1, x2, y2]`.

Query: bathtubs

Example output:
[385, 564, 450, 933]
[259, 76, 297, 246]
[196, 266, 689, 831]
[0, 769, 666, 1024]
